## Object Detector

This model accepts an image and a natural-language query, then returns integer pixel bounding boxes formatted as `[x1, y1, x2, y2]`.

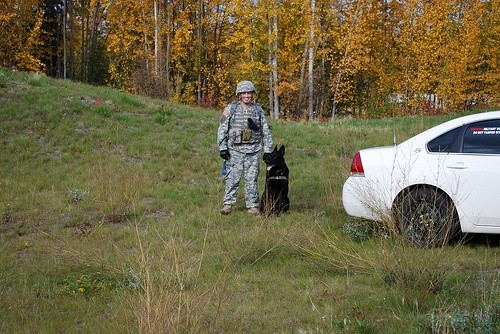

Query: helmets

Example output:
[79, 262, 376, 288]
[235, 80, 258, 96]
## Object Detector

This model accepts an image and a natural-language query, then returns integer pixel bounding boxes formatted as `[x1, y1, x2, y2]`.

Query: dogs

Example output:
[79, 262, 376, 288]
[261, 144, 290, 218]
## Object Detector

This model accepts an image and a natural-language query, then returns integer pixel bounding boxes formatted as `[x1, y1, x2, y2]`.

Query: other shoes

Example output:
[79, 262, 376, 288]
[221, 204, 232, 215]
[247, 207, 261, 218]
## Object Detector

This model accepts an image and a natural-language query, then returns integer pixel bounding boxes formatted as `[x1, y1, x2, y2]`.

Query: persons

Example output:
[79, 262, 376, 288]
[217, 80, 272, 214]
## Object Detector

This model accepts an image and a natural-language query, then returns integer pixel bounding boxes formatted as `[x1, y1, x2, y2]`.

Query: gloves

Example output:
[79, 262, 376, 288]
[263, 153, 270, 161]
[219, 149, 230, 160]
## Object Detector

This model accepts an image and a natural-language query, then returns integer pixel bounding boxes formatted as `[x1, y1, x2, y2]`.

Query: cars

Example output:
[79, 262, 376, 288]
[341, 110, 500, 247]
